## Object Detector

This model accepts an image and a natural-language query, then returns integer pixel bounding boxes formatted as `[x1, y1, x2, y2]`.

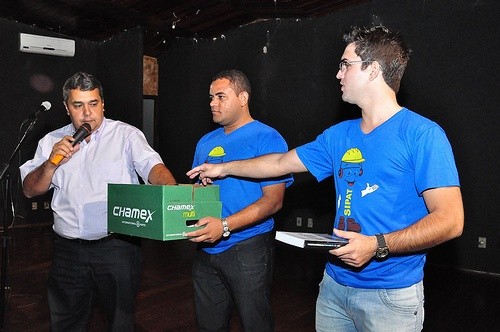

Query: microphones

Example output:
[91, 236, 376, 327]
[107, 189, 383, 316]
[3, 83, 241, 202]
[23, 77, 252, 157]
[24, 101, 52, 122]
[50, 122, 91, 166]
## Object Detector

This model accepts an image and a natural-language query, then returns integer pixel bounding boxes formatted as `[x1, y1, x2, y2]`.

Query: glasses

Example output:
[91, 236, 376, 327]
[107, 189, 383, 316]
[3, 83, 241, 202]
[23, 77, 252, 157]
[339, 59, 383, 73]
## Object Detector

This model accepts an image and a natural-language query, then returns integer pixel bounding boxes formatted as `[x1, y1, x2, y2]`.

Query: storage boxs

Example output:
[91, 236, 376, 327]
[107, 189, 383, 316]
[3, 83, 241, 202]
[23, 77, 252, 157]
[108, 183, 222, 241]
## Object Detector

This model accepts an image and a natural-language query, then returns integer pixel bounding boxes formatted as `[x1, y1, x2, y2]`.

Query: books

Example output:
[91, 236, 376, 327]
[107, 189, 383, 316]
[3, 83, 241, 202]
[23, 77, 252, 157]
[275, 231, 349, 249]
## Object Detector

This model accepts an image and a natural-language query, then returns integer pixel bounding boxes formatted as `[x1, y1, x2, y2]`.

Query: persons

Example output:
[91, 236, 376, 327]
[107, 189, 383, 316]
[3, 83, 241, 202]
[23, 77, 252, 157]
[186, 25, 464, 332]
[19, 72, 176, 332]
[185, 69, 294, 332]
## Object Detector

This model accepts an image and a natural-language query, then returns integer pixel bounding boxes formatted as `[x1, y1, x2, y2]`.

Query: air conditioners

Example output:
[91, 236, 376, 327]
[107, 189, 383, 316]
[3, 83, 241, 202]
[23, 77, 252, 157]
[19, 33, 75, 57]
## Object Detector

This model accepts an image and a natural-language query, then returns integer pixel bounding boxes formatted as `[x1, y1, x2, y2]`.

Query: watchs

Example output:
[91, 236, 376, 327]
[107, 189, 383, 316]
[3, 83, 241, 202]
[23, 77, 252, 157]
[374, 233, 390, 260]
[220, 218, 230, 237]
